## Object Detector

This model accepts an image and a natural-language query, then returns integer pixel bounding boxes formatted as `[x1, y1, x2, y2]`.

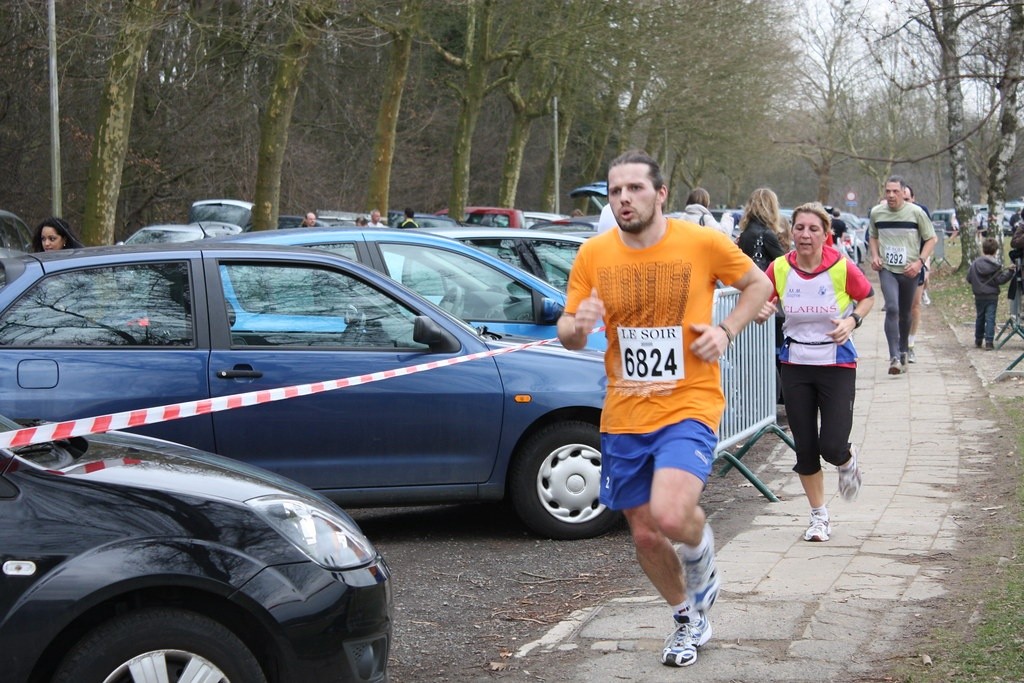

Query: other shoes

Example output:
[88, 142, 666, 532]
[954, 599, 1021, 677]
[900, 352, 907, 372]
[907, 335, 916, 348]
[888, 357, 901, 375]
[922, 293, 931, 305]
[976, 339, 982, 346]
[985, 343, 994, 351]
[882, 304, 887, 311]
[907, 347, 916, 363]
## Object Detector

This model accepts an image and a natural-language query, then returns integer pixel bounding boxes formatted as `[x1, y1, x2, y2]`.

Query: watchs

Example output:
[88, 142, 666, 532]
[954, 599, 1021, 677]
[848, 312, 863, 331]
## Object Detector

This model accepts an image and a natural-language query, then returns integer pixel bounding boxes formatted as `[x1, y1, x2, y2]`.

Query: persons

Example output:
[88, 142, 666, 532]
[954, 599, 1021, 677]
[33, 218, 93, 314]
[571, 209, 583, 218]
[558, 148, 775, 667]
[401, 207, 418, 228]
[831, 210, 846, 244]
[719, 322, 735, 348]
[966, 208, 1024, 351]
[947, 212, 959, 246]
[753, 202, 876, 543]
[355, 216, 369, 227]
[302, 212, 320, 227]
[737, 188, 792, 273]
[678, 187, 734, 240]
[864, 175, 931, 374]
[368, 209, 383, 228]
[598, 202, 618, 234]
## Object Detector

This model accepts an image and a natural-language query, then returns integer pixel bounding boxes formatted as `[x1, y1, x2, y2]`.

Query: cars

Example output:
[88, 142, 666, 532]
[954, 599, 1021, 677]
[0, 249, 626, 540]
[0, 413, 393, 683]
[0, 209, 35, 249]
[112, 183, 793, 270]
[826, 206, 871, 265]
[193, 229, 612, 357]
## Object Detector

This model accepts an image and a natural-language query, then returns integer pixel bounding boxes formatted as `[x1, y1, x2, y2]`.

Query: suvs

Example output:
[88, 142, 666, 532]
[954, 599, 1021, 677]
[931, 203, 1024, 238]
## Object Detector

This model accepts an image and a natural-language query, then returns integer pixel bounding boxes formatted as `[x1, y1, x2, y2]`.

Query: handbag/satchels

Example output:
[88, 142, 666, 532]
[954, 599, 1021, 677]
[751, 228, 770, 273]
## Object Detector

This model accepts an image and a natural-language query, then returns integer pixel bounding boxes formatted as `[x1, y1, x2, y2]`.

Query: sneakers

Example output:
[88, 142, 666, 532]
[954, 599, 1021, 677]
[803, 509, 831, 543]
[838, 443, 862, 502]
[678, 522, 721, 611]
[661, 610, 711, 667]
